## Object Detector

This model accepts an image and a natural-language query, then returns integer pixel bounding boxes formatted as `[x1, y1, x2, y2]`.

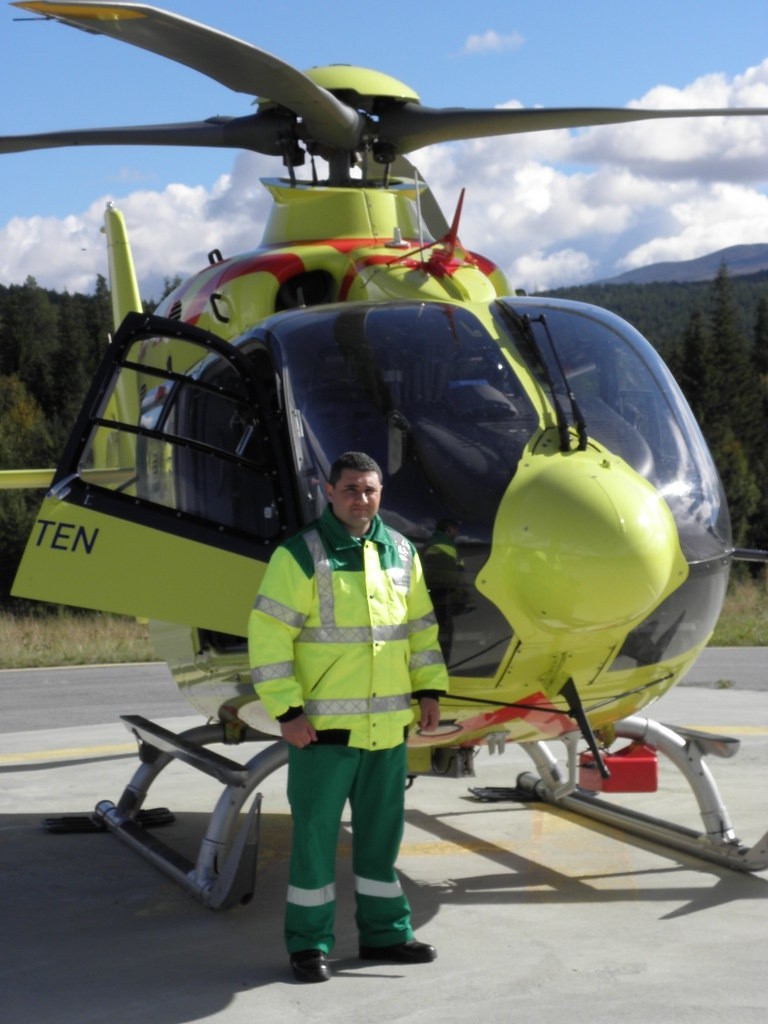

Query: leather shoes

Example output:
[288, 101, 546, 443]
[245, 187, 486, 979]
[290, 949, 331, 982]
[359, 938, 437, 963]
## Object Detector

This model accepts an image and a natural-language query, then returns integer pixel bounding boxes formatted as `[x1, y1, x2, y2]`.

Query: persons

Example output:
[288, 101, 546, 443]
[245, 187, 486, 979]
[248, 451, 451, 981]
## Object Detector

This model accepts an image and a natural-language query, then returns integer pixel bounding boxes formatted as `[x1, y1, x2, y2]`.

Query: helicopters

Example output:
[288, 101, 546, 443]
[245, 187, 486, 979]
[0, 0, 767, 910]
